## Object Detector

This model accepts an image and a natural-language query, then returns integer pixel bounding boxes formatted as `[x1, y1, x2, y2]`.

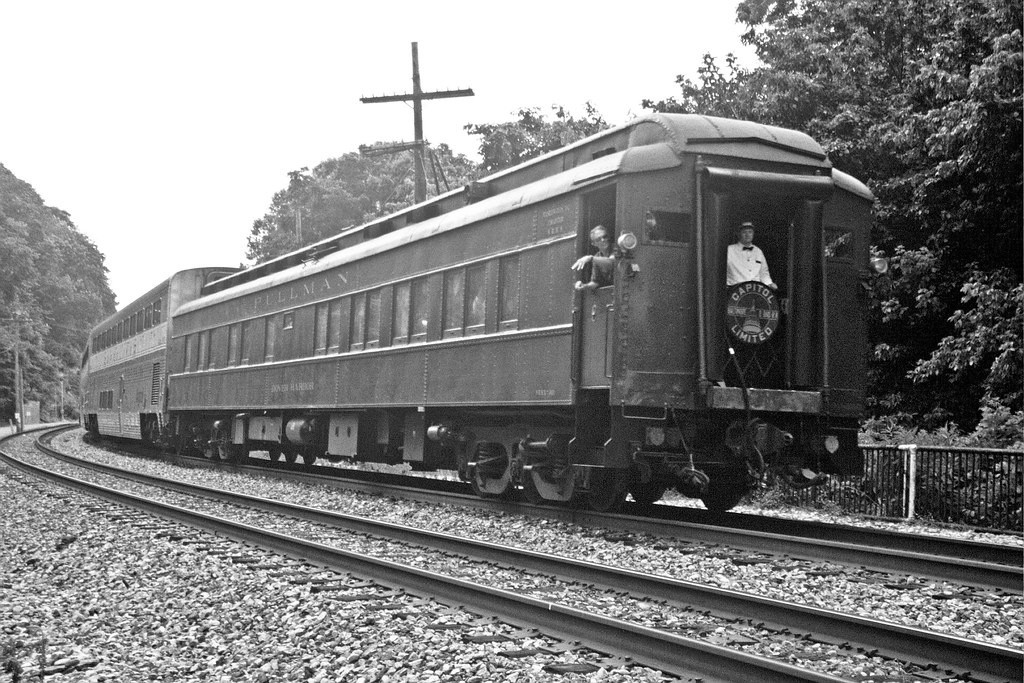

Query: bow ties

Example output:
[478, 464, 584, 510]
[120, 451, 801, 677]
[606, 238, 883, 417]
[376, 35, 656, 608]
[743, 246, 753, 251]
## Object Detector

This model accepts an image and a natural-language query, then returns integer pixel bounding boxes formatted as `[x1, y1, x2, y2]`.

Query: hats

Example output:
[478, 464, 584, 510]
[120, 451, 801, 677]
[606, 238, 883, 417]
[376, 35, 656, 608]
[739, 222, 755, 229]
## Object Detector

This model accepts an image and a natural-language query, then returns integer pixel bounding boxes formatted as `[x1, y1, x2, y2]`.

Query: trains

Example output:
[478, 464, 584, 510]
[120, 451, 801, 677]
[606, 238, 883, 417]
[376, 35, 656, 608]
[79, 112, 888, 512]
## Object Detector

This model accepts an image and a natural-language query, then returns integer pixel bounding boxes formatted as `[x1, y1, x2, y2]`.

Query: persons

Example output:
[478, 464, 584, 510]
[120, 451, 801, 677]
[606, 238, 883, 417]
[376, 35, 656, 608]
[723, 220, 779, 309]
[571, 225, 615, 291]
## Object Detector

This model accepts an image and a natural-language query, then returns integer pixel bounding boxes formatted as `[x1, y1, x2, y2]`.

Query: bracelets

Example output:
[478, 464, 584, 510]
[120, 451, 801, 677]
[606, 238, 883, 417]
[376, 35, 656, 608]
[589, 255, 593, 261]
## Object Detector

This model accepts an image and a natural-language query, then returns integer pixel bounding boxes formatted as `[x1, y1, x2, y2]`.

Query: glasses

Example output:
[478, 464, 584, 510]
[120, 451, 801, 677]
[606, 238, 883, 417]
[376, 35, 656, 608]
[595, 233, 609, 242]
